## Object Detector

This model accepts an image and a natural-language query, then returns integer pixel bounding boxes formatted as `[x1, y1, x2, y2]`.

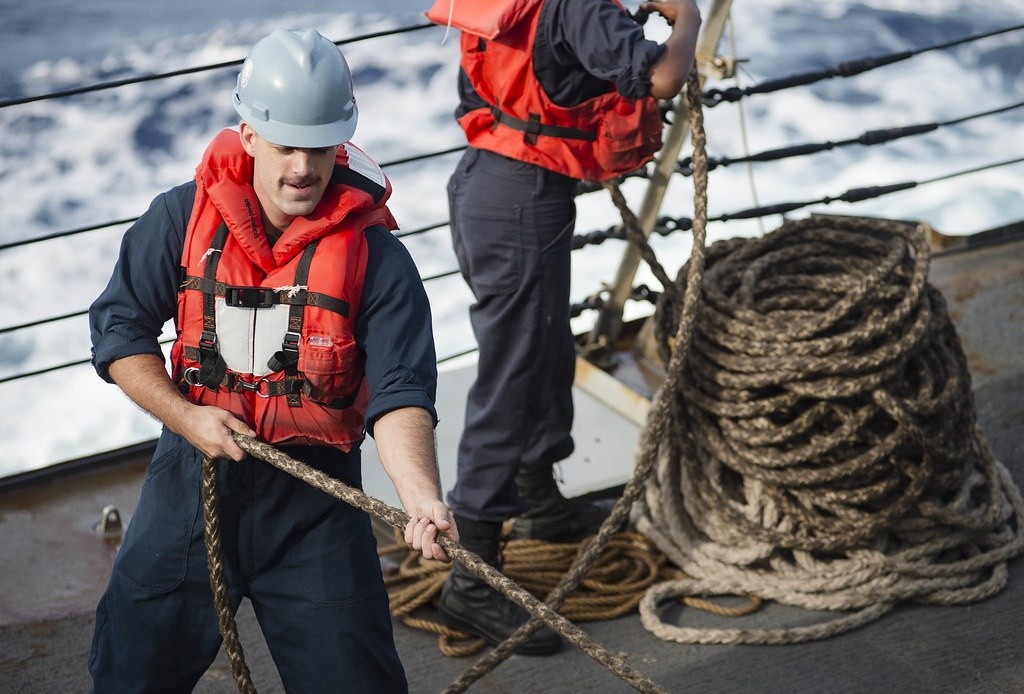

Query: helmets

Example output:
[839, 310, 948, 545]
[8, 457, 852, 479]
[231, 27, 358, 148]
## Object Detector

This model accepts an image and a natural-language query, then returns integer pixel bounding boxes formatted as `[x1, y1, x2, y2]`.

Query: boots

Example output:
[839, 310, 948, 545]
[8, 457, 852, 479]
[438, 514, 563, 655]
[510, 468, 609, 543]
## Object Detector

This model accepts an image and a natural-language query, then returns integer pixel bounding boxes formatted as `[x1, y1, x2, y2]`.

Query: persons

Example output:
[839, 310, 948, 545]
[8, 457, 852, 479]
[89, 30, 462, 693]
[440, 0, 702, 656]
